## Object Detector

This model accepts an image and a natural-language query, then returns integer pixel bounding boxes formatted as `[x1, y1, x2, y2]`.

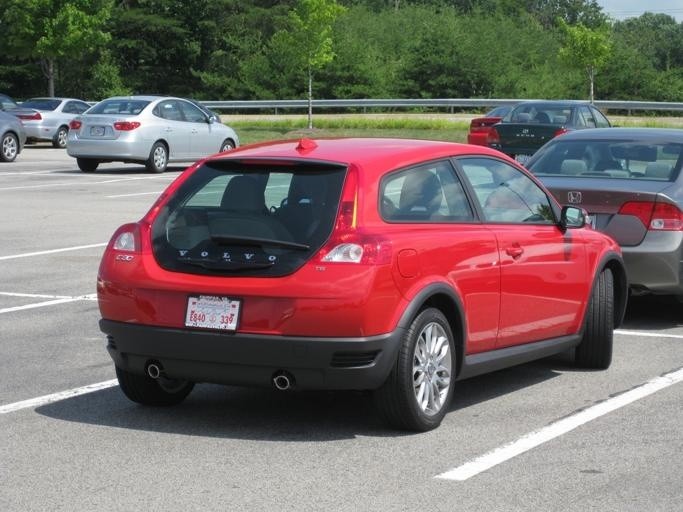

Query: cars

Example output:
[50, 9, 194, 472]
[0, 94, 241, 173]
[468, 98, 622, 161]
[484, 126, 683, 298]
[96, 135, 630, 434]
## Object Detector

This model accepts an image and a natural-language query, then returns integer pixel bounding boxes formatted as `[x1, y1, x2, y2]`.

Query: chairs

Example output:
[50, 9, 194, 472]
[552, 115, 567, 125]
[516, 113, 529, 123]
[390, 169, 445, 220]
[559, 159, 586, 175]
[644, 162, 672, 179]
[205, 175, 296, 253]
